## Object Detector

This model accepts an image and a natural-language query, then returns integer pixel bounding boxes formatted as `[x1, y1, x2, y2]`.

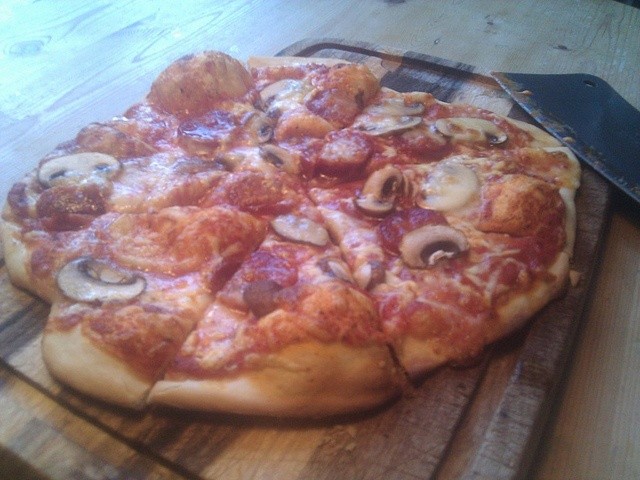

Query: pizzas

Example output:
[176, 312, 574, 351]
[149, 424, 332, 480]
[1, 48, 583, 420]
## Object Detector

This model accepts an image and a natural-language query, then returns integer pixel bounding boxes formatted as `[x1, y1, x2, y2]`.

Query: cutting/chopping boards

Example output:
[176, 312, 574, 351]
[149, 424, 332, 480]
[2, 33, 616, 480]
